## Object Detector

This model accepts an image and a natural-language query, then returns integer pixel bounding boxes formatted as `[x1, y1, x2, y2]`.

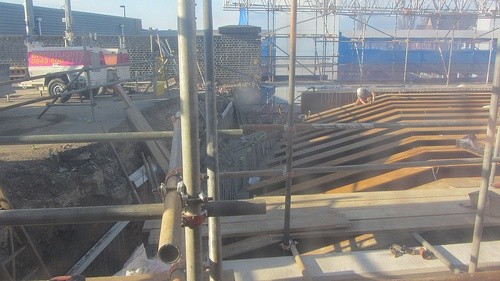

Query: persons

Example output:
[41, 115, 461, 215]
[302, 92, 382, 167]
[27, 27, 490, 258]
[355, 87, 376, 106]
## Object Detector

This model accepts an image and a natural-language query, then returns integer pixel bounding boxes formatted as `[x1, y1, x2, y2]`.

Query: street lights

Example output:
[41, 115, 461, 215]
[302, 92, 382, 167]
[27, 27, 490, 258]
[119, 5, 127, 36]
